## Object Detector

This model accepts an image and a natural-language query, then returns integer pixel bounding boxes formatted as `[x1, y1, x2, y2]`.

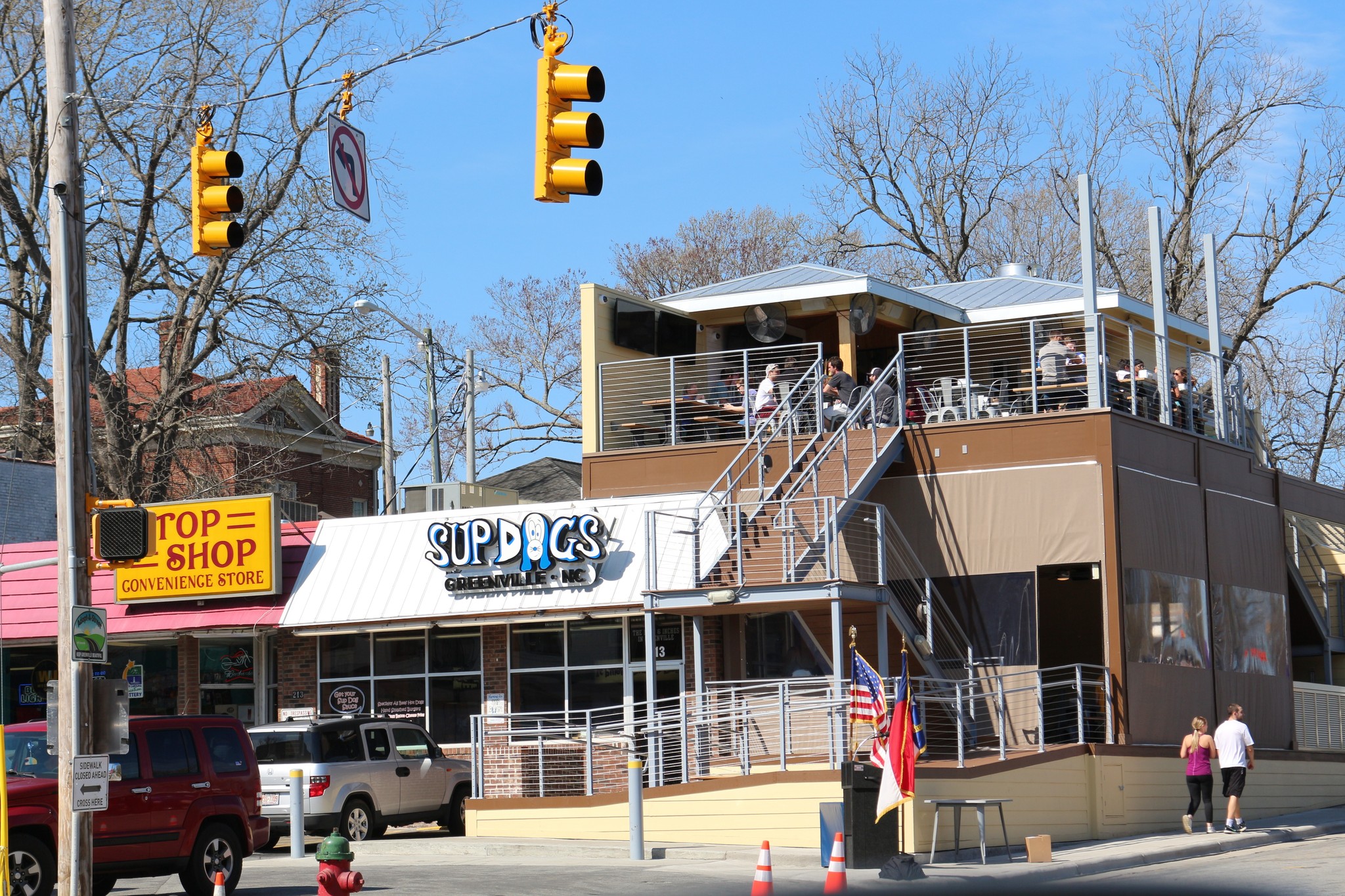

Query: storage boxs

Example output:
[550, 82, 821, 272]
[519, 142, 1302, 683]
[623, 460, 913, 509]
[1025, 833, 1054, 863]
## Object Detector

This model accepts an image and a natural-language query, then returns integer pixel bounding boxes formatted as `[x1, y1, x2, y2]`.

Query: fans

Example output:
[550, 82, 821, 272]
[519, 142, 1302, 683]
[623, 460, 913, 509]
[1020, 316, 1062, 353]
[742, 302, 788, 343]
[910, 308, 941, 359]
[848, 292, 878, 336]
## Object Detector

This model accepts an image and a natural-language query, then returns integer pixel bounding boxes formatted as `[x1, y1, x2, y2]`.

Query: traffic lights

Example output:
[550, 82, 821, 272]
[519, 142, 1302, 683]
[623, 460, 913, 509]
[100, 508, 157, 560]
[534, 57, 605, 203]
[191, 145, 245, 257]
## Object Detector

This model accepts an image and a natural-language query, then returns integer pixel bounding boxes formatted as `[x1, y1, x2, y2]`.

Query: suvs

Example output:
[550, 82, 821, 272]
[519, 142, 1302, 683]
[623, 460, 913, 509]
[3, 713, 271, 896]
[246, 712, 479, 853]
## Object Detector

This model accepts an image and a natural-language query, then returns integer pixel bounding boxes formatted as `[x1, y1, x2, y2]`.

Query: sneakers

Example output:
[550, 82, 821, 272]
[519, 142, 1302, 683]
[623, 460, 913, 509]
[1224, 825, 1240, 834]
[1183, 815, 1192, 834]
[1206, 826, 1216, 833]
[1238, 821, 1247, 832]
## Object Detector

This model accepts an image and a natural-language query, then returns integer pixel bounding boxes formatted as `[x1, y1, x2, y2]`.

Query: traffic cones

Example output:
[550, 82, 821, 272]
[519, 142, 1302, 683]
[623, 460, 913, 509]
[213, 872, 225, 896]
[750, 840, 775, 896]
[822, 833, 847, 896]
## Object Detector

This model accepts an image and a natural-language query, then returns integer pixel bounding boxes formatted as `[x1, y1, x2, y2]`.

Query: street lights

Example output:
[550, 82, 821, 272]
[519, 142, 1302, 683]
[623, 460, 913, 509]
[353, 299, 442, 483]
[466, 349, 489, 484]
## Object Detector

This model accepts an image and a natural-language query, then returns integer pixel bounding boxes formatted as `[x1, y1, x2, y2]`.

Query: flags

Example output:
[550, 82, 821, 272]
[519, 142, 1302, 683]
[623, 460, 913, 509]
[875, 649, 926, 825]
[850, 644, 891, 769]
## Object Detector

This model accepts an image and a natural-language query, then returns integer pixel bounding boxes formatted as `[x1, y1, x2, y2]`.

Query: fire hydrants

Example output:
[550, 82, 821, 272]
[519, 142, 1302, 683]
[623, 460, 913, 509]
[315, 828, 365, 896]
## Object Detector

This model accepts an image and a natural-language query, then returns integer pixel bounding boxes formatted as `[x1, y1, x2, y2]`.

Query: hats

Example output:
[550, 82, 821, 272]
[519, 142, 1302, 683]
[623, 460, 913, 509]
[720, 369, 737, 376]
[765, 364, 779, 378]
[867, 368, 883, 376]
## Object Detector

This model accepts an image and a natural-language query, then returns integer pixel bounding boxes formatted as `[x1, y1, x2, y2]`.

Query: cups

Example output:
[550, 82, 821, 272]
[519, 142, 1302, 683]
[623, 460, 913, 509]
[713, 401, 720, 407]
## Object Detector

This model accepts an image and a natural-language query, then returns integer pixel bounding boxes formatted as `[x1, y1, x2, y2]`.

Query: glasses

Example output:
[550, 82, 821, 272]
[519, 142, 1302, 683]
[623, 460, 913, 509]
[1136, 365, 1146, 369]
[1061, 336, 1064, 338]
[1172, 373, 1181, 375]
[737, 385, 742, 389]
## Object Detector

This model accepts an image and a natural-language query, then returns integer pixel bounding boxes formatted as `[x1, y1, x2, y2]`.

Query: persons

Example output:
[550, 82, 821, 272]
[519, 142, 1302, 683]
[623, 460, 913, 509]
[753, 364, 785, 434]
[679, 383, 715, 442]
[705, 368, 734, 440]
[1038, 329, 1083, 413]
[858, 367, 896, 429]
[1153, 365, 1182, 428]
[905, 372, 932, 424]
[1058, 336, 1088, 409]
[810, 357, 859, 431]
[1116, 359, 1146, 417]
[721, 378, 757, 438]
[1215, 704, 1255, 833]
[1181, 716, 1216, 834]
[1172, 367, 1205, 435]
[772, 358, 815, 435]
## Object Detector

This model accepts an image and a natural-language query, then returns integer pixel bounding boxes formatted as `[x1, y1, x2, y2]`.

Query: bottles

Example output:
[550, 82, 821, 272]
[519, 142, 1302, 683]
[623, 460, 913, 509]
[1037, 356, 1041, 368]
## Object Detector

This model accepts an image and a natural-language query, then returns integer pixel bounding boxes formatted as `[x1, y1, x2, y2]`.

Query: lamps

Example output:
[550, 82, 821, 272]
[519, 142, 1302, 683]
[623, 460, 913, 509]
[1125, 317, 1144, 333]
[1073, 311, 1084, 320]
[706, 591, 738, 605]
[877, 301, 904, 319]
[800, 296, 832, 312]
[913, 633, 933, 660]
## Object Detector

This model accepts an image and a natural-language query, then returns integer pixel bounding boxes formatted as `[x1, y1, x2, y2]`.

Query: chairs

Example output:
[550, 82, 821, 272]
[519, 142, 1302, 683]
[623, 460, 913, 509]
[972, 378, 1009, 418]
[771, 381, 812, 435]
[916, 387, 941, 423]
[828, 385, 869, 432]
[933, 377, 966, 421]
[866, 394, 898, 429]
[747, 394, 777, 437]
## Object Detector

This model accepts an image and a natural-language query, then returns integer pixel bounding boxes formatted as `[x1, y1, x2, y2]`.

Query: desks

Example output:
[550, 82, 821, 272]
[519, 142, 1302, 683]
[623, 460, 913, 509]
[1019, 363, 1087, 406]
[929, 383, 1000, 422]
[643, 398, 742, 445]
[924, 795, 1013, 864]
[803, 387, 840, 432]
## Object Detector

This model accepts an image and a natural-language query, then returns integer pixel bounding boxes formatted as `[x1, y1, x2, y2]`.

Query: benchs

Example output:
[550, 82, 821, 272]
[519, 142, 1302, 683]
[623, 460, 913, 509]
[1012, 381, 1087, 411]
[620, 422, 666, 443]
[695, 414, 773, 434]
[1112, 389, 1208, 434]
